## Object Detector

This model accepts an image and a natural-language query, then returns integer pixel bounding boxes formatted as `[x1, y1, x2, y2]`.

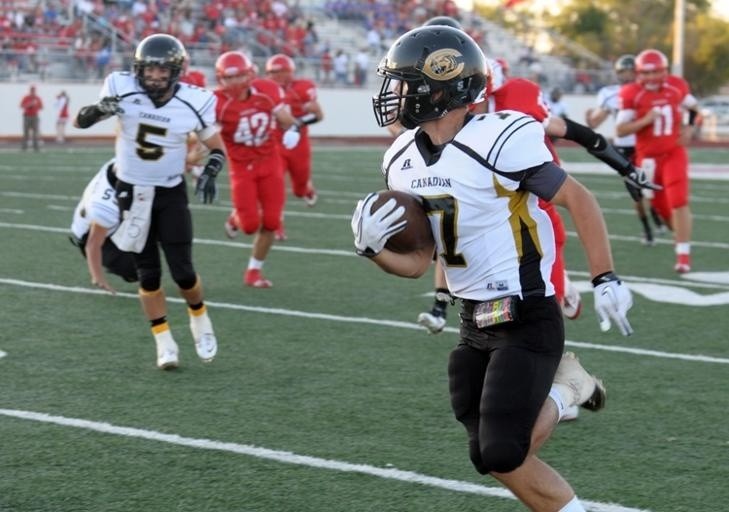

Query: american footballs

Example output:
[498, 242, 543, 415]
[362, 190, 434, 251]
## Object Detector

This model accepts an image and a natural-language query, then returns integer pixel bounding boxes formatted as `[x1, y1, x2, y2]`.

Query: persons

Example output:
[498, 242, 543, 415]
[19, 86, 43, 151]
[388, 15, 463, 333]
[54, 90, 71, 145]
[471, 59, 665, 319]
[263, 53, 324, 206]
[350, 22, 631, 512]
[66, 32, 227, 371]
[585, 53, 663, 246]
[617, 51, 702, 273]
[187, 52, 301, 289]
[180, 70, 209, 179]
[70, 141, 209, 294]
[2, 1, 600, 102]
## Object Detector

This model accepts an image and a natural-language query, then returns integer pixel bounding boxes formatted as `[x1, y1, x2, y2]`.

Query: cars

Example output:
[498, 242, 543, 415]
[692, 94, 729, 145]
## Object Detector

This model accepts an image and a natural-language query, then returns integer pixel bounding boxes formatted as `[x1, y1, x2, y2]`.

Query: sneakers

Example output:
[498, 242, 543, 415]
[417, 308, 447, 336]
[675, 255, 691, 273]
[154, 329, 179, 369]
[190, 310, 217, 360]
[553, 351, 607, 411]
[225, 209, 239, 239]
[246, 270, 273, 288]
[304, 181, 317, 206]
[560, 269, 581, 320]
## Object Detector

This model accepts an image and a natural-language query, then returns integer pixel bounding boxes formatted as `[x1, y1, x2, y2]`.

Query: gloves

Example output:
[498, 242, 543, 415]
[351, 192, 409, 258]
[283, 123, 301, 150]
[78, 96, 124, 128]
[593, 272, 633, 336]
[194, 149, 225, 204]
[620, 162, 664, 202]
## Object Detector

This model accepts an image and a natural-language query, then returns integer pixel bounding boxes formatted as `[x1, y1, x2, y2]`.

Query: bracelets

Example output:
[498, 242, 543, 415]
[693, 115, 701, 125]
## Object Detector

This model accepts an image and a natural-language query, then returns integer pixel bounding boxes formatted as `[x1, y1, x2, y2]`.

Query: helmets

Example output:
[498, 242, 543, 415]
[134, 33, 187, 100]
[373, 25, 488, 126]
[266, 53, 296, 73]
[634, 49, 669, 91]
[614, 54, 635, 85]
[215, 51, 259, 88]
